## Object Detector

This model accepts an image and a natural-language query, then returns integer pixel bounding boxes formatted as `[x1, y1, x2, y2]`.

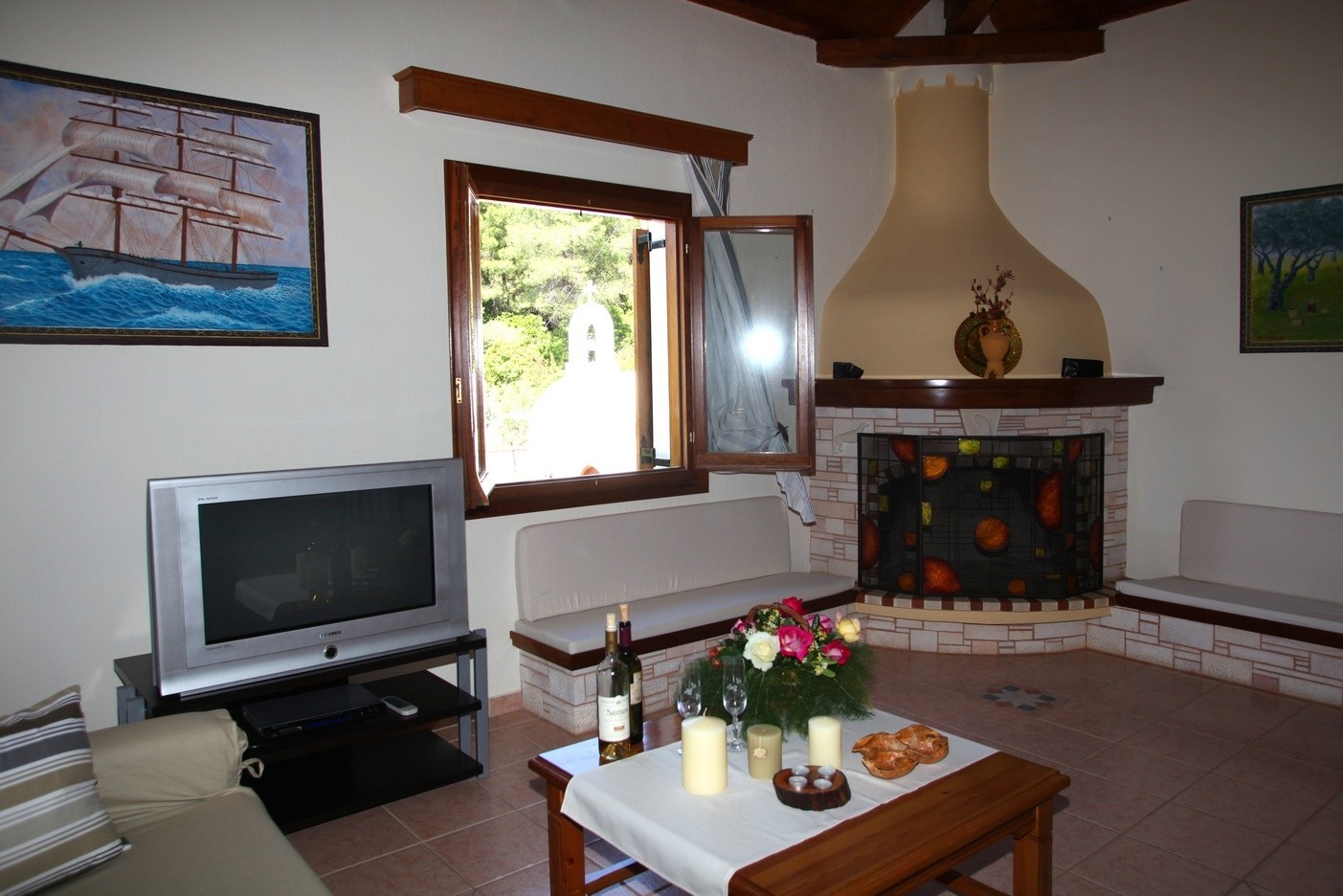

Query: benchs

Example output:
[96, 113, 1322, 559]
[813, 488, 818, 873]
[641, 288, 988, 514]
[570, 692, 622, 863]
[1114, 499, 1342, 711]
[508, 495, 859, 738]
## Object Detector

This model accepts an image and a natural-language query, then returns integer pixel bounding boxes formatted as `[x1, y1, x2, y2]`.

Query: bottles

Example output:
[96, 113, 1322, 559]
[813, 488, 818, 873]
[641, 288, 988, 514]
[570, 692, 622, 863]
[616, 603, 643, 743]
[596, 612, 631, 759]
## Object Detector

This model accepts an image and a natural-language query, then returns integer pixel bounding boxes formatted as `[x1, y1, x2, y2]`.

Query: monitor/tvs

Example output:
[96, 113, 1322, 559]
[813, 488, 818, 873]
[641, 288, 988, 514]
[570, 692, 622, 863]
[147, 454, 471, 700]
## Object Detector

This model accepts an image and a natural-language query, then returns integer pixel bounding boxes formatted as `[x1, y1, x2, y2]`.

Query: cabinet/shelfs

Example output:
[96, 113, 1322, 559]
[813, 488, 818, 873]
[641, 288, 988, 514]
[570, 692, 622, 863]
[113, 627, 487, 836]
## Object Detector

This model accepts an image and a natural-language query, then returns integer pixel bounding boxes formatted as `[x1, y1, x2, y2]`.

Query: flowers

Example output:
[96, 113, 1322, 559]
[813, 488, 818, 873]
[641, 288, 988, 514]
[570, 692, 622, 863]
[674, 595, 874, 744]
[970, 264, 1017, 324]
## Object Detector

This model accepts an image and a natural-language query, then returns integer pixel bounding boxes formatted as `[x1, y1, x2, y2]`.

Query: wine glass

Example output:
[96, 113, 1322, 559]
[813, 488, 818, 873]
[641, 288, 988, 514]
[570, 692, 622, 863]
[677, 660, 702, 753]
[723, 657, 747, 752]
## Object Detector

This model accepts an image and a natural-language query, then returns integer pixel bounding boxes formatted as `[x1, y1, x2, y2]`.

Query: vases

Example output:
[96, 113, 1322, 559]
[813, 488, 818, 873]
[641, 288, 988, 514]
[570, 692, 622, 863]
[978, 319, 1012, 380]
[746, 602, 815, 640]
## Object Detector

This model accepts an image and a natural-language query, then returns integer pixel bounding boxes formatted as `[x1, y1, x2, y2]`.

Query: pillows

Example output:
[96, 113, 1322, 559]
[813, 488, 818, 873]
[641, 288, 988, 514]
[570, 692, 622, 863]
[0, 683, 136, 896]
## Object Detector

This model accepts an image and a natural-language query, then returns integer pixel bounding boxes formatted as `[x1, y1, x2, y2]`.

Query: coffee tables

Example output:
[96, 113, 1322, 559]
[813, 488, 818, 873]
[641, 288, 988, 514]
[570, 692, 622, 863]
[527, 701, 1071, 896]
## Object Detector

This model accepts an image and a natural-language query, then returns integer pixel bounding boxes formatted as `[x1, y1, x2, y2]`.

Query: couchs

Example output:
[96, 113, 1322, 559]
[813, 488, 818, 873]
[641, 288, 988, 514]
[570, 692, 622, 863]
[31, 707, 335, 896]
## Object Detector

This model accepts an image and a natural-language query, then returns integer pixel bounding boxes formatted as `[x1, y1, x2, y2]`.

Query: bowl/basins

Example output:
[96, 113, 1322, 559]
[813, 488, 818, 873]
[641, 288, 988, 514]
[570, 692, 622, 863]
[818, 766, 837, 777]
[792, 764, 810, 777]
[789, 777, 807, 787]
[814, 779, 831, 789]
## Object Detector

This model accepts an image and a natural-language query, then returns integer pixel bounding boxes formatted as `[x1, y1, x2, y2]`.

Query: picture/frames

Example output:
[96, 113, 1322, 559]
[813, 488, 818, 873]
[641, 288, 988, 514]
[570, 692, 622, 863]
[1, 58, 328, 347]
[1239, 183, 1343, 353]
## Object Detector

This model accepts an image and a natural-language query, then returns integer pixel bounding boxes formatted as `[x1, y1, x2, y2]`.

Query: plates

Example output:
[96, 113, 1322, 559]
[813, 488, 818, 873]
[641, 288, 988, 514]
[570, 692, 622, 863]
[850, 724, 950, 780]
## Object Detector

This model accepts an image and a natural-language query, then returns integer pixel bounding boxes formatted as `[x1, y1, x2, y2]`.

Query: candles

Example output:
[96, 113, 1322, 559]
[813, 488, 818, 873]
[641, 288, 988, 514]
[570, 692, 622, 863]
[807, 716, 842, 771]
[788, 776, 808, 789]
[681, 708, 729, 797]
[746, 724, 782, 780]
[791, 765, 810, 777]
[817, 765, 836, 779]
[813, 778, 833, 789]
